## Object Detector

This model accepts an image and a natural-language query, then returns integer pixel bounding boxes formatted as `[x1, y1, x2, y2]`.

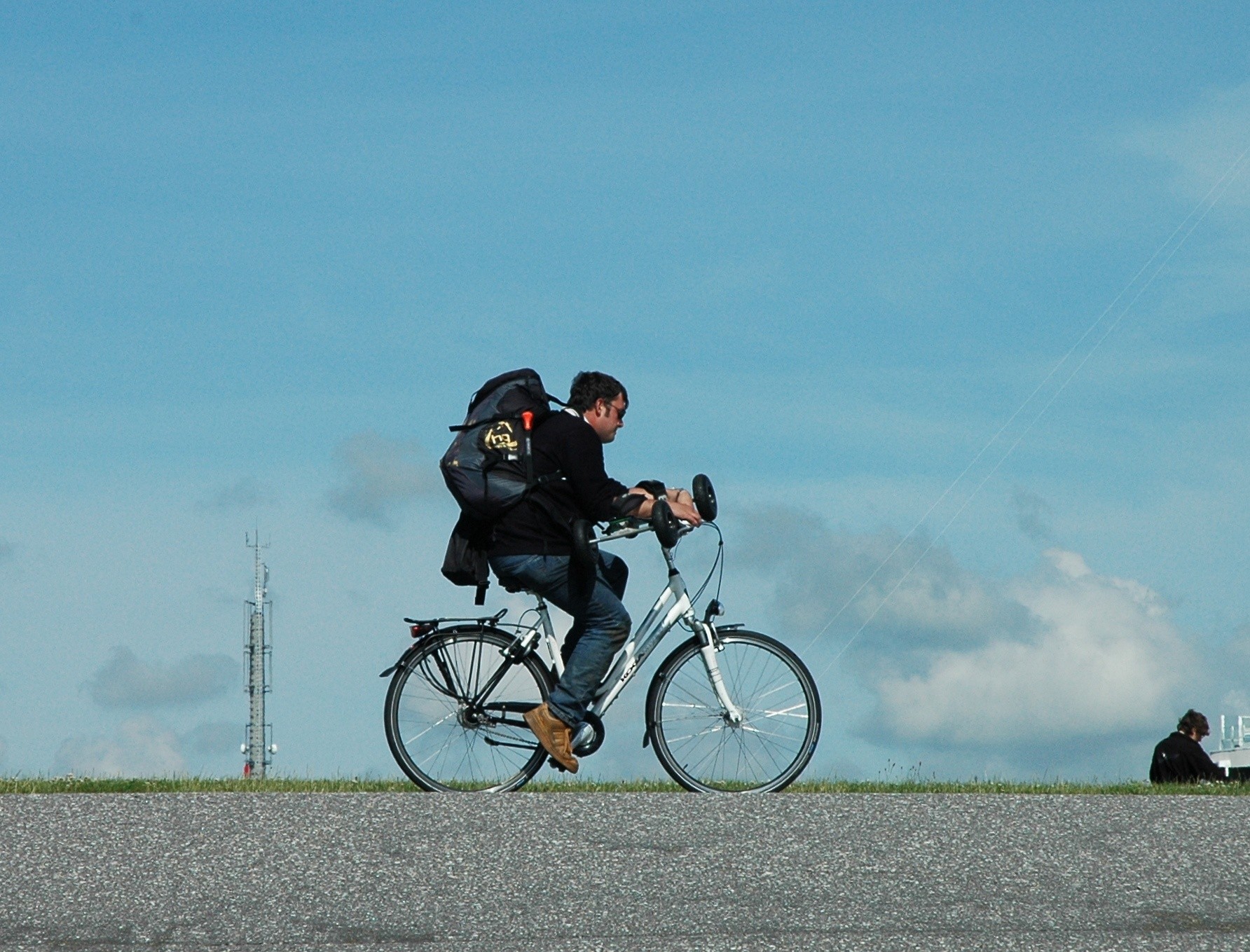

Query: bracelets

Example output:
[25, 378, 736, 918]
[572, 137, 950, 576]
[674, 488, 682, 504]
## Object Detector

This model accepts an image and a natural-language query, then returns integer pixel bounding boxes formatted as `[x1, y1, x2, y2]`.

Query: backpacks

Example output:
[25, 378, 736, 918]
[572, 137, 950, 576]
[439, 368, 583, 514]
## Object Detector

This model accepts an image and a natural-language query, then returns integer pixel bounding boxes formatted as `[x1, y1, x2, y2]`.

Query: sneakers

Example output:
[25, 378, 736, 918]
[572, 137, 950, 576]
[523, 702, 579, 774]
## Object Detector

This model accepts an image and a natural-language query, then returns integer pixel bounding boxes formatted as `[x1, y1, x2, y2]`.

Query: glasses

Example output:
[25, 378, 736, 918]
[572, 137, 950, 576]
[606, 401, 626, 420]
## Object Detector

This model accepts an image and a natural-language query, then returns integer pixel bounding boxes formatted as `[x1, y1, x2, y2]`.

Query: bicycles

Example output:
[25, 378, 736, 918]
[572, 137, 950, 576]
[378, 474, 823, 796]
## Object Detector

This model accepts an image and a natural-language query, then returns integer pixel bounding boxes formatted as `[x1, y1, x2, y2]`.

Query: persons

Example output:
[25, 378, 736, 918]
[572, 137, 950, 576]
[487, 372, 701, 774]
[1149, 710, 1230, 784]
